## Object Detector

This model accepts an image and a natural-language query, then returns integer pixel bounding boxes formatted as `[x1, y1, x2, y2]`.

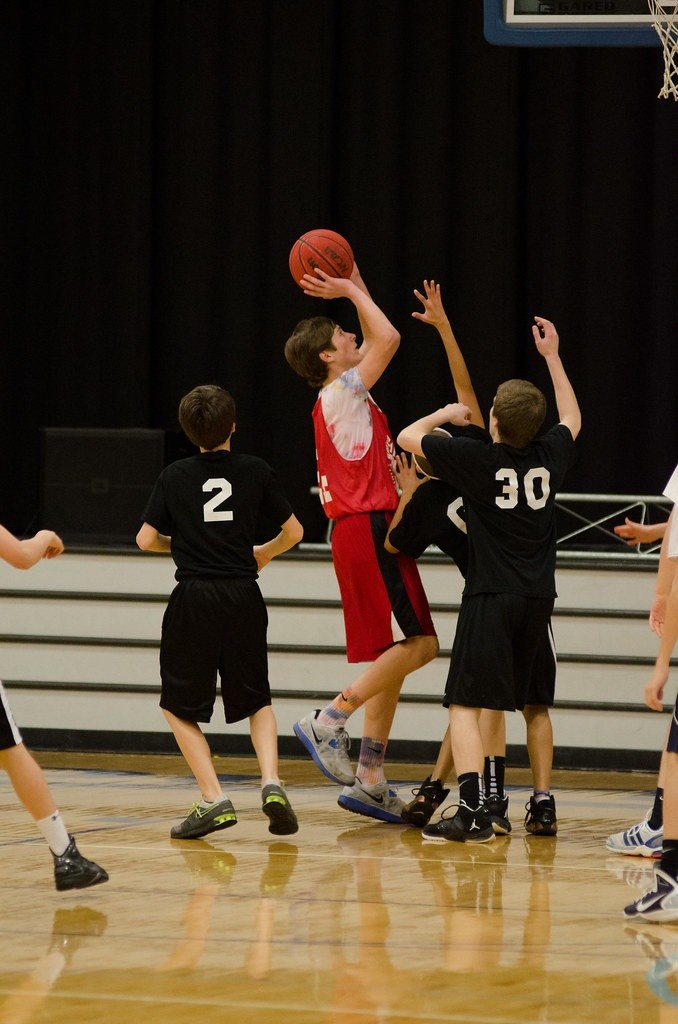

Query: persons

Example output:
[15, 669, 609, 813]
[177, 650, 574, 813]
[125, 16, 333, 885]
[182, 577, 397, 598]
[137, 386, 298, 841]
[384, 280, 510, 834]
[606, 466, 678, 922]
[286, 262, 439, 822]
[396, 315, 582, 843]
[0, 527, 109, 893]
[0, 828, 678, 1024]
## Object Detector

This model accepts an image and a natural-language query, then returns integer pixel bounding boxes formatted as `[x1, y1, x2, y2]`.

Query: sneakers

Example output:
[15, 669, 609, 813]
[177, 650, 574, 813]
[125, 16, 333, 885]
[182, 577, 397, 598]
[401, 773, 451, 827]
[338, 775, 407, 822]
[606, 806, 664, 857]
[622, 923, 678, 982]
[293, 707, 356, 785]
[172, 838, 237, 887]
[48, 908, 108, 964]
[482, 792, 511, 835]
[48, 834, 108, 891]
[337, 824, 558, 878]
[524, 792, 556, 836]
[262, 780, 298, 835]
[623, 868, 678, 922]
[603, 859, 658, 894]
[421, 802, 495, 843]
[259, 843, 299, 899]
[170, 799, 236, 838]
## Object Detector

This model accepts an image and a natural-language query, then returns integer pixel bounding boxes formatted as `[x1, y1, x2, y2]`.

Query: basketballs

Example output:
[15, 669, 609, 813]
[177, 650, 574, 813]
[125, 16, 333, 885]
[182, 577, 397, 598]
[287, 227, 354, 297]
